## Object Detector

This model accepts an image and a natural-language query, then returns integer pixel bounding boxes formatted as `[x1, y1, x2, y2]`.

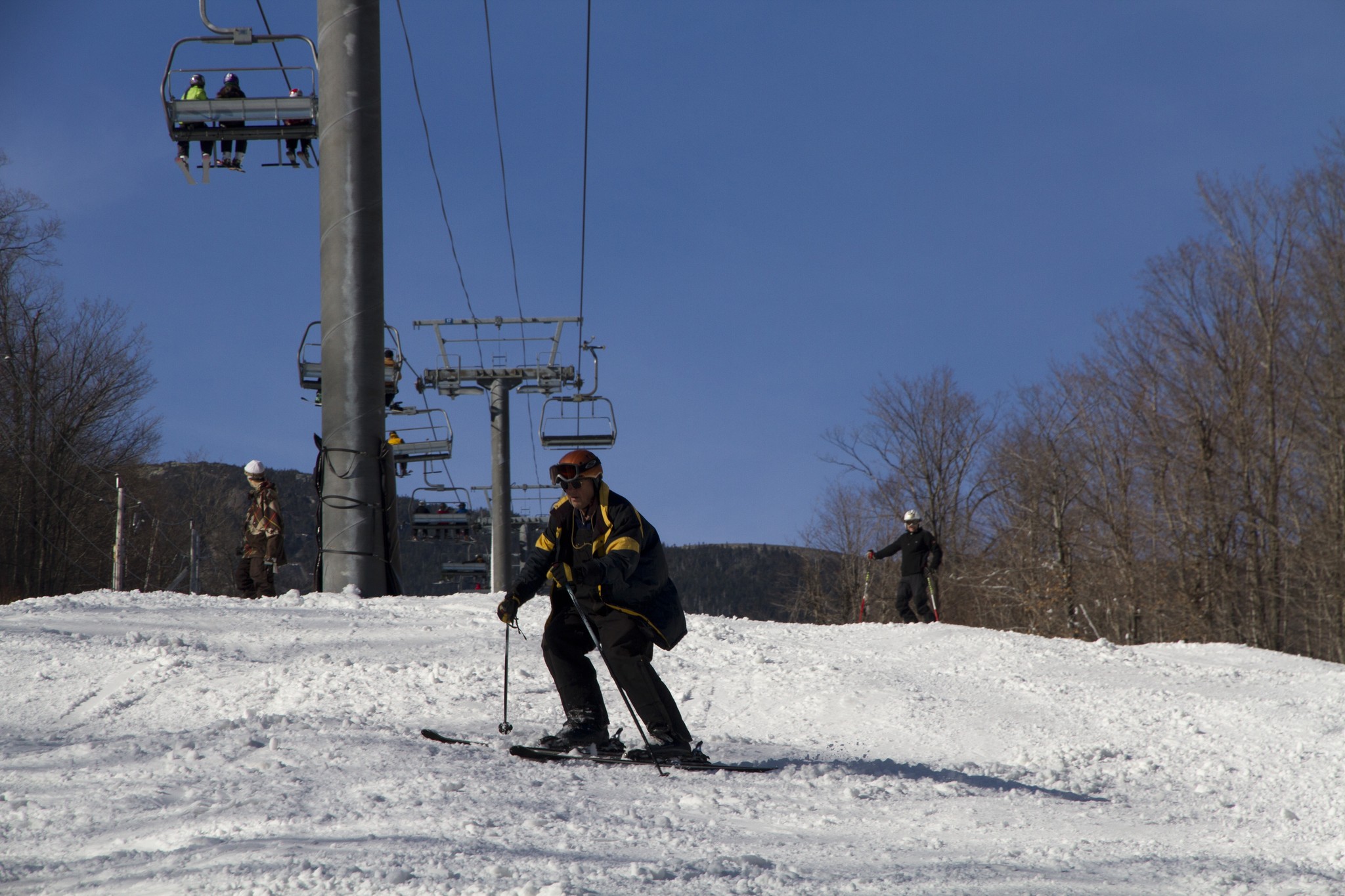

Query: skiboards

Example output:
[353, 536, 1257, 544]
[300, 396, 322, 406]
[173, 152, 211, 186]
[406, 537, 477, 546]
[285, 149, 314, 169]
[421, 729, 780, 774]
[396, 470, 413, 478]
[214, 158, 246, 173]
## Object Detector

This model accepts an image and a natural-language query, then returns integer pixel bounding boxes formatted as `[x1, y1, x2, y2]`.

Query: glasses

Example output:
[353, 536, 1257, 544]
[560, 479, 593, 490]
[906, 520, 919, 525]
[549, 463, 577, 485]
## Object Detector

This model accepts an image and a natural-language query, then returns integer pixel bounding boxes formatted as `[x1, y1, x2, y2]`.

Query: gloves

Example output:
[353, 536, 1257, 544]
[497, 596, 520, 623]
[865, 549, 876, 560]
[263, 556, 273, 563]
[923, 567, 934, 578]
[546, 562, 572, 587]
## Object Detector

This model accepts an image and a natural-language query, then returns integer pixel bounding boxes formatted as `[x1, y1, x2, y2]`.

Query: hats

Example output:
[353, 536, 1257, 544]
[244, 460, 265, 479]
[390, 431, 396, 437]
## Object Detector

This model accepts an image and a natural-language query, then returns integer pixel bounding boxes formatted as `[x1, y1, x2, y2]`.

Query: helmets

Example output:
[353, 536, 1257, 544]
[904, 509, 922, 522]
[557, 450, 603, 479]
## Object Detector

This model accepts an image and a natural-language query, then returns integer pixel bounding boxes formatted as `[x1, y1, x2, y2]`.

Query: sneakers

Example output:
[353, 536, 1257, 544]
[625, 741, 692, 760]
[538, 723, 609, 751]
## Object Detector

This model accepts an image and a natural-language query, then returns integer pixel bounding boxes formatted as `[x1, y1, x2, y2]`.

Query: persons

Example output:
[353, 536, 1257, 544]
[177, 74, 214, 168]
[867, 509, 943, 623]
[455, 503, 469, 541]
[384, 350, 401, 406]
[386, 431, 408, 475]
[216, 73, 247, 168]
[441, 561, 455, 581]
[497, 449, 693, 763]
[472, 554, 486, 583]
[235, 460, 287, 600]
[435, 503, 451, 539]
[412, 501, 429, 541]
[282, 88, 310, 160]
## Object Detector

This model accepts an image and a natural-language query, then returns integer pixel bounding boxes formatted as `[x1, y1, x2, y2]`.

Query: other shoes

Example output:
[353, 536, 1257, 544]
[315, 393, 322, 406]
[390, 404, 405, 412]
[403, 469, 407, 474]
